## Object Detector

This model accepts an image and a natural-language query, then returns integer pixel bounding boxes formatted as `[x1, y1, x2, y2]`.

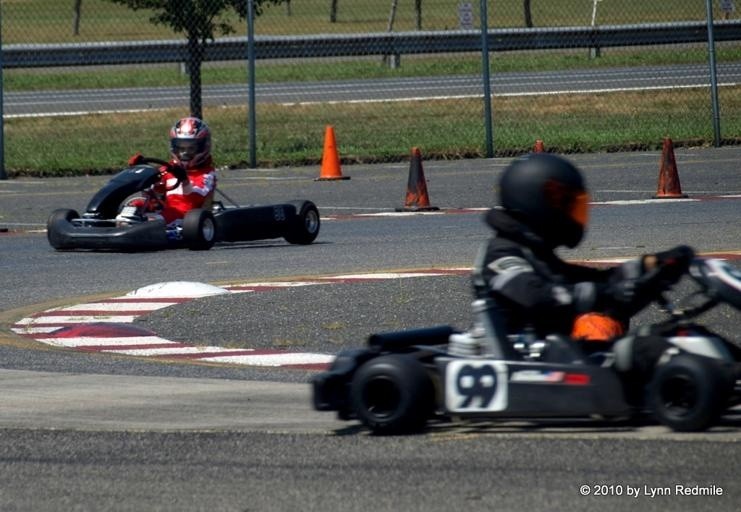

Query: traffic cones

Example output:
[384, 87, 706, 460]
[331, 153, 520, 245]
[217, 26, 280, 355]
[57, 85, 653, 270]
[534, 140, 545, 153]
[313, 124, 351, 183]
[651, 134, 689, 201]
[394, 145, 439, 214]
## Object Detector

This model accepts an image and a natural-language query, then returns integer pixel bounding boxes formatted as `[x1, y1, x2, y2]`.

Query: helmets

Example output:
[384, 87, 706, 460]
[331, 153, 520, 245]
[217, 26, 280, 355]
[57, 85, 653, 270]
[499, 152, 591, 249]
[169, 117, 211, 170]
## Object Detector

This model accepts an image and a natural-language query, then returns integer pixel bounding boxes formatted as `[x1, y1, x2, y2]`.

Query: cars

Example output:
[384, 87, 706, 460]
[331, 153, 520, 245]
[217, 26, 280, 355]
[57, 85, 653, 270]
[312, 256, 741, 435]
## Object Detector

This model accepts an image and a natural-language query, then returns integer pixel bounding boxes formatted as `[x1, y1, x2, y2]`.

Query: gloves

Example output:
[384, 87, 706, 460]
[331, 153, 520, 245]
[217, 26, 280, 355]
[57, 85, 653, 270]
[655, 245, 693, 275]
[594, 278, 637, 312]
[128, 152, 149, 167]
[166, 163, 189, 183]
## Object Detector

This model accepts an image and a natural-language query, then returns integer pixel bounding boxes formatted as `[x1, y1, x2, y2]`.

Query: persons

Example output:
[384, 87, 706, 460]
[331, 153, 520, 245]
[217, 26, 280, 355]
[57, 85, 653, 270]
[471, 152, 665, 366]
[114, 117, 217, 230]
